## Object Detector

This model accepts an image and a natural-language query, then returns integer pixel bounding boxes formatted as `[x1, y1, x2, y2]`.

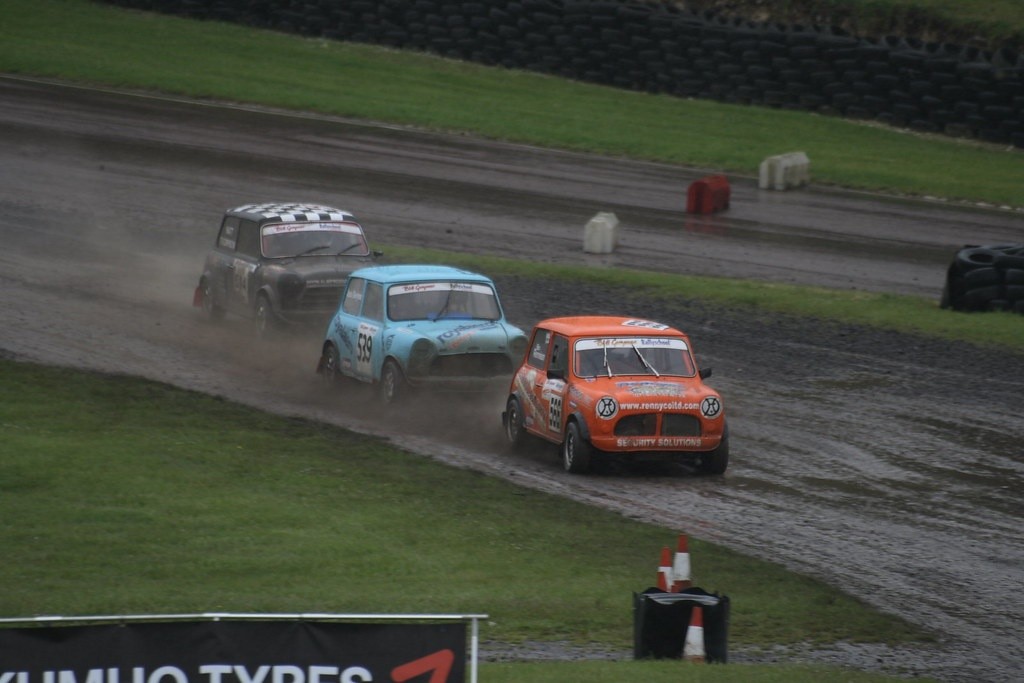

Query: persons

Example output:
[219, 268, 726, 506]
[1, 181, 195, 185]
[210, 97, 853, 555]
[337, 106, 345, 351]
[445, 291, 473, 313]
[646, 350, 685, 374]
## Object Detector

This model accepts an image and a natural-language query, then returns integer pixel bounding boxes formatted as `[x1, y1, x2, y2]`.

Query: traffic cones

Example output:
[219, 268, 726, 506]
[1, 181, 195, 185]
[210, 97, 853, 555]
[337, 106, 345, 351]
[657, 548, 674, 595]
[671, 530, 692, 596]
[679, 605, 708, 662]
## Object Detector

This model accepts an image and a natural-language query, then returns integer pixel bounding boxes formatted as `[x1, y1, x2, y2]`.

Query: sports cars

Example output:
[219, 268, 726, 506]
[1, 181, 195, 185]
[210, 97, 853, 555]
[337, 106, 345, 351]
[500, 315, 730, 476]
[314, 264, 534, 424]
[190, 201, 387, 342]
